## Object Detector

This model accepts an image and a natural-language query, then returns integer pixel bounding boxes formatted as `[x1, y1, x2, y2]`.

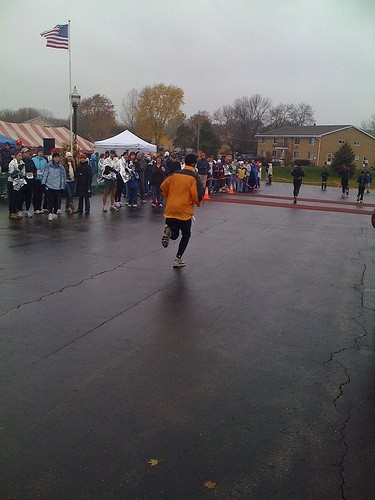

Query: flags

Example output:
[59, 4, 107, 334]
[39, 24, 69, 49]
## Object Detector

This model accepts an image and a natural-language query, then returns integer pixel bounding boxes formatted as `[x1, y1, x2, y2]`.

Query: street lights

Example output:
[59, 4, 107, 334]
[68, 85, 81, 173]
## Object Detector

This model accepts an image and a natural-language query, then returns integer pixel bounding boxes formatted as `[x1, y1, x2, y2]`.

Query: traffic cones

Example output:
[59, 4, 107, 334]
[228, 184, 235, 195]
[203, 187, 211, 200]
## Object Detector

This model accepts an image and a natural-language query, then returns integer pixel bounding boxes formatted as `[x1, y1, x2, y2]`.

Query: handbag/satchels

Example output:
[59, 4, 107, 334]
[12, 173, 27, 191]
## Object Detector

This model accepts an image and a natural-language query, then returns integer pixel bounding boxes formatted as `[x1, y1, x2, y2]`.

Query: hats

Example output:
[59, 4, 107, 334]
[21, 148, 30, 153]
[51, 147, 57, 153]
[80, 154, 86, 159]
[65, 151, 73, 157]
[37, 147, 44, 153]
[15, 149, 22, 156]
[16, 139, 22, 145]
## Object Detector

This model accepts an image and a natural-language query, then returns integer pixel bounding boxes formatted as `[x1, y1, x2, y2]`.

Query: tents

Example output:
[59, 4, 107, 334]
[93, 129, 158, 159]
[0, 120, 95, 153]
[0, 134, 17, 147]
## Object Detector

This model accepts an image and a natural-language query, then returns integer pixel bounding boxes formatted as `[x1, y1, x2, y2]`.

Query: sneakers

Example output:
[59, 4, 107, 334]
[173, 257, 186, 268]
[161, 226, 171, 248]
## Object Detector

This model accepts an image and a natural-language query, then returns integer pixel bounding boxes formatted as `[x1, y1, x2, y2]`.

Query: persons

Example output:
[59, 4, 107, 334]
[292, 163, 305, 204]
[365, 171, 372, 193]
[321, 168, 329, 192]
[0, 140, 272, 221]
[356, 170, 367, 203]
[160, 154, 204, 267]
[339, 163, 353, 198]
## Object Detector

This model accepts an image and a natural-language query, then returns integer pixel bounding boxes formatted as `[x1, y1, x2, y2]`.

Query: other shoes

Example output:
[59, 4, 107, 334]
[110, 205, 117, 210]
[115, 199, 163, 209]
[47, 213, 57, 220]
[103, 206, 107, 212]
[9, 208, 62, 219]
